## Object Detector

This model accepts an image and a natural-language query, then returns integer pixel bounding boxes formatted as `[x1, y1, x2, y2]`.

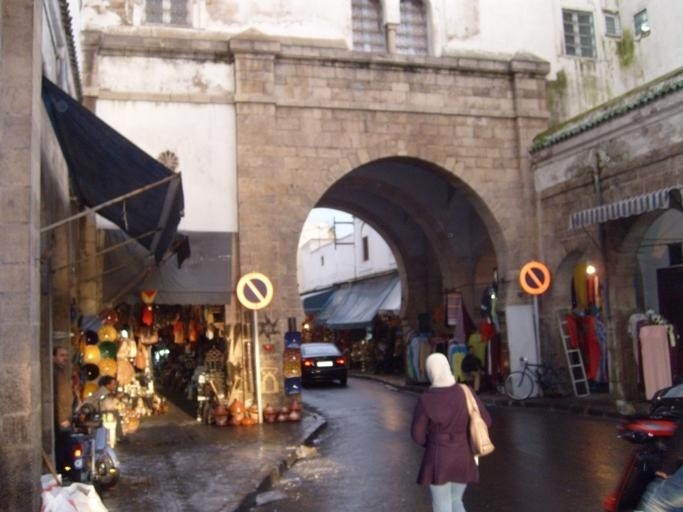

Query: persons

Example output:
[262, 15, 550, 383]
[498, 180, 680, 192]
[51, 344, 81, 482]
[460, 344, 491, 395]
[409, 351, 492, 512]
[83, 375, 128, 444]
[637, 425, 682, 512]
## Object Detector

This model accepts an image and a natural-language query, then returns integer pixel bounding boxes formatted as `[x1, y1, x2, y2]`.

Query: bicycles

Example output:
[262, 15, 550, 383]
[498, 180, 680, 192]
[504, 350, 569, 401]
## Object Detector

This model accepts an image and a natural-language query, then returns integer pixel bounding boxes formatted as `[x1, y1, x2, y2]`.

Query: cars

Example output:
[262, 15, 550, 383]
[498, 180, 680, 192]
[602, 383, 683, 512]
[300, 342, 348, 389]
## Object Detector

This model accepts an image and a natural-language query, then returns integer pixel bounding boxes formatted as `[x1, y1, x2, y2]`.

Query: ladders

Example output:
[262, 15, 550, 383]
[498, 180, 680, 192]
[555, 305, 591, 399]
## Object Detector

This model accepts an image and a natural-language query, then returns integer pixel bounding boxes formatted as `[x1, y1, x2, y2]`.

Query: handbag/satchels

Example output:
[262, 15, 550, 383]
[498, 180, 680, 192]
[459, 383, 496, 459]
